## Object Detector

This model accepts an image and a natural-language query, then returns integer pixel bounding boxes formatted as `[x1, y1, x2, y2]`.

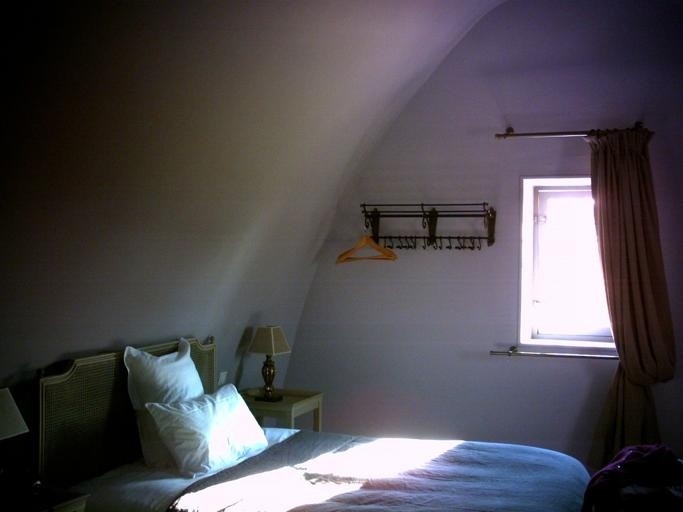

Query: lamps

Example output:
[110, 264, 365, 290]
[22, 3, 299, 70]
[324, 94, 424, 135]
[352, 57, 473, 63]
[248, 325, 292, 401]
[0, 387, 30, 440]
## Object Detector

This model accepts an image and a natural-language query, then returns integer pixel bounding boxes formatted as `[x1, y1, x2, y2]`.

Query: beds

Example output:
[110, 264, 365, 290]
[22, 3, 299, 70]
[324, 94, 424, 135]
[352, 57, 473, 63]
[37, 336, 593, 512]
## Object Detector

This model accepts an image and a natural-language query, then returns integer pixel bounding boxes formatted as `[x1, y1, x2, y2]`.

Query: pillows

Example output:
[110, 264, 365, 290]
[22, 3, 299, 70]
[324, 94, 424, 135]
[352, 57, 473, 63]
[124, 338, 204, 475]
[145, 384, 268, 478]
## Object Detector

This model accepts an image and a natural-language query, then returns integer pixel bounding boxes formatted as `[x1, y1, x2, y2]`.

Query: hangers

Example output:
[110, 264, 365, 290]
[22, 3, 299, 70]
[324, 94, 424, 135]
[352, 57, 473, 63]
[337, 217, 396, 263]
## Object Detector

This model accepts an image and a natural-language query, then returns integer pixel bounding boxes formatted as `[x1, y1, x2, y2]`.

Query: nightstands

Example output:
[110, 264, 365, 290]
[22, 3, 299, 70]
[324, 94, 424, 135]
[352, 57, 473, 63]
[239, 389, 323, 432]
[15, 485, 90, 512]
